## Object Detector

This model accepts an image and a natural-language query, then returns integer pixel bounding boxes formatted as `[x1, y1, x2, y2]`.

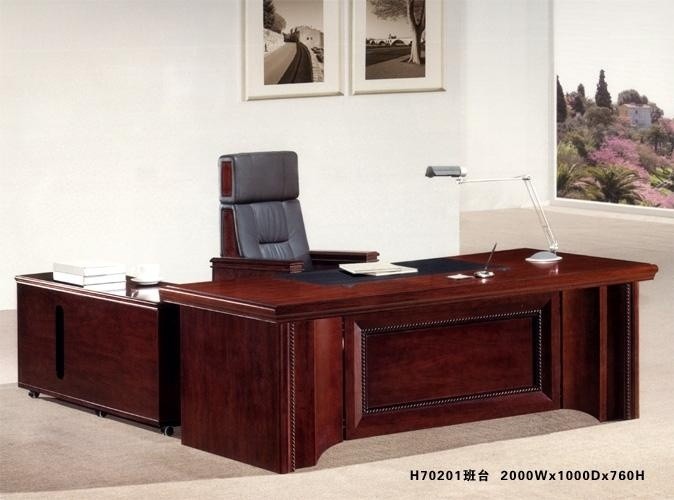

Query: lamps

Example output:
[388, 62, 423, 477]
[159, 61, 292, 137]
[422, 163, 564, 263]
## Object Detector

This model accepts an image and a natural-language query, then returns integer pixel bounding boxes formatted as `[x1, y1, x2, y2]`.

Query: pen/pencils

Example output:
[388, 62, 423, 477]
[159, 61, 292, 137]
[485, 243, 497, 270]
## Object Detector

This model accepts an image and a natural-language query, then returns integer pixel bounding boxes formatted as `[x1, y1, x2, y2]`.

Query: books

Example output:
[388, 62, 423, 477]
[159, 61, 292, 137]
[338, 262, 419, 277]
[53, 262, 126, 286]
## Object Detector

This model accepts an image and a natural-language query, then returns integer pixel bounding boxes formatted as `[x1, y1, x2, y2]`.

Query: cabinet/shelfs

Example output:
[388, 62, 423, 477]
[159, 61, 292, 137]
[13, 268, 181, 436]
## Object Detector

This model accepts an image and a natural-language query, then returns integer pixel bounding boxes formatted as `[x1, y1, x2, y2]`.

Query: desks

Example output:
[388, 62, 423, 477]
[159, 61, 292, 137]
[156, 244, 660, 474]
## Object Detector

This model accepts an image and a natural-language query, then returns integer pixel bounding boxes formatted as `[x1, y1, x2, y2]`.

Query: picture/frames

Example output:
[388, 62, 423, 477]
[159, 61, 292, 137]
[238, 0, 346, 104]
[544, 15, 674, 226]
[346, 0, 447, 97]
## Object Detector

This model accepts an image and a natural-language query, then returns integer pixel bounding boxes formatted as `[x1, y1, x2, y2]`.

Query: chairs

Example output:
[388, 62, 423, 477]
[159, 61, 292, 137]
[210, 150, 381, 282]
